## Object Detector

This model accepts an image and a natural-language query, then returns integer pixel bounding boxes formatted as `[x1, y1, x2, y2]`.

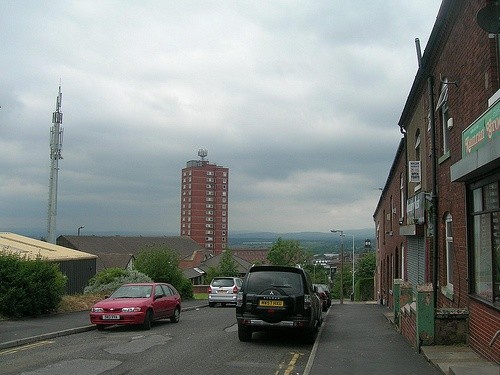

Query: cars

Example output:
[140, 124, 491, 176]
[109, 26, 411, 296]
[90, 283, 182, 330]
[208, 277, 243, 307]
[312, 282, 332, 310]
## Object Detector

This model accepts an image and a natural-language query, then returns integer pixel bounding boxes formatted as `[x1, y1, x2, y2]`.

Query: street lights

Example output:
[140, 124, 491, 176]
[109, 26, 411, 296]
[340, 234, 354, 293]
[330, 230, 344, 304]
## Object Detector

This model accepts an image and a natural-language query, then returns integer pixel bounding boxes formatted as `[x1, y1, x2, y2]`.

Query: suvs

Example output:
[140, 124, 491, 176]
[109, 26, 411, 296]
[235, 264, 323, 341]
